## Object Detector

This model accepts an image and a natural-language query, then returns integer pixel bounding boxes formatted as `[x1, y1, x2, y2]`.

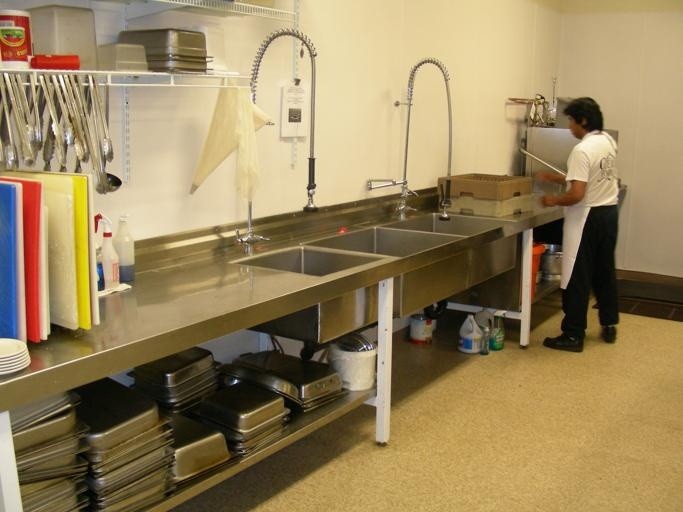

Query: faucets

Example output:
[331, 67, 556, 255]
[366, 56, 453, 220]
[247, 26, 320, 213]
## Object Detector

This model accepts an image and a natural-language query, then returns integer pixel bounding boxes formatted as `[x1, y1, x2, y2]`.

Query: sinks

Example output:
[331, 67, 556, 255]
[301, 226, 468, 259]
[376, 209, 520, 240]
[227, 244, 390, 277]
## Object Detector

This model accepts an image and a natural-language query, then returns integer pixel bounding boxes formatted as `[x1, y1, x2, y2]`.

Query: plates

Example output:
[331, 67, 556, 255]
[0, 337, 31, 376]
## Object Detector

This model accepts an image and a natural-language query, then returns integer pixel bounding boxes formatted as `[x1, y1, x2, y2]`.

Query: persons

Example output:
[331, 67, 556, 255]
[534, 96, 622, 351]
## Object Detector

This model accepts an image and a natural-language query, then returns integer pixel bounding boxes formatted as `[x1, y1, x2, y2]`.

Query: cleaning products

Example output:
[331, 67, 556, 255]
[94, 214, 120, 291]
[490, 317, 506, 349]
[482, 326, 490, 355]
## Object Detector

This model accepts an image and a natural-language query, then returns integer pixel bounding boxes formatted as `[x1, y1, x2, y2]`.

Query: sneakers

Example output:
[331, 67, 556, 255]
[543, 333, 585, 352]
[602, 326, 616, 343]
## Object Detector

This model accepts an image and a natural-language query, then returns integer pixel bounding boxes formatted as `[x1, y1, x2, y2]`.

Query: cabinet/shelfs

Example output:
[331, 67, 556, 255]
[10, 1, 300, 90]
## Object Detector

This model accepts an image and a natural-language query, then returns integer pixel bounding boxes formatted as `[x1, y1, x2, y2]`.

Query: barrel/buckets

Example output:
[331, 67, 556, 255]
[408, 314, 438, 344]
[325, 342, 378, 393]
[457, 314, 481, 354]
[531, 243, 545, 304]
[474, 307, 496, 332]
[540, 243, 564, 275]
